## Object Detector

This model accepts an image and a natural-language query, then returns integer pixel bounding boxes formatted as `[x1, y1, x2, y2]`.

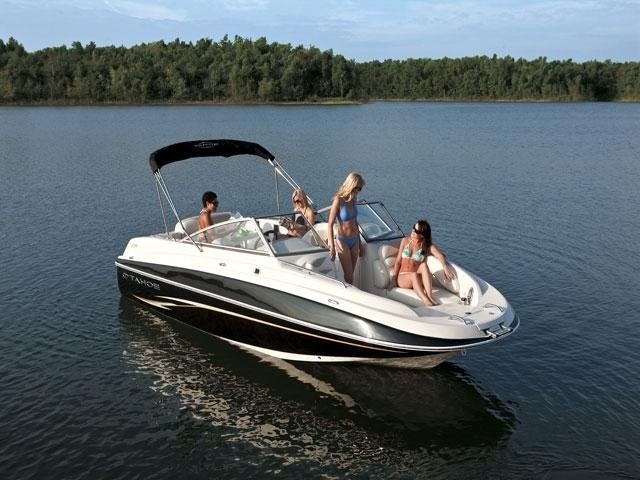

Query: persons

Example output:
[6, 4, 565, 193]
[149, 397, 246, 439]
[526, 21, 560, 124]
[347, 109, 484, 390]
[328, 171, 365, 285]
[197, 191, 234, 243]
[391, 219, 456, 306]
[272, 188, 314, 241]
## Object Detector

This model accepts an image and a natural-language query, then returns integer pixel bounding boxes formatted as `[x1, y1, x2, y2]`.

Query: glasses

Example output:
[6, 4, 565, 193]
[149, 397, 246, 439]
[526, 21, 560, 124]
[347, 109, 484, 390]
[211, 201, 219, 206]
[413, 224, 420, 234]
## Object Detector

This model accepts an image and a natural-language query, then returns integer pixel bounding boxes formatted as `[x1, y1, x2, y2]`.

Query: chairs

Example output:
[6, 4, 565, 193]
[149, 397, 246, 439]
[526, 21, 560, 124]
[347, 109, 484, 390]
[378, 244, 462, 308]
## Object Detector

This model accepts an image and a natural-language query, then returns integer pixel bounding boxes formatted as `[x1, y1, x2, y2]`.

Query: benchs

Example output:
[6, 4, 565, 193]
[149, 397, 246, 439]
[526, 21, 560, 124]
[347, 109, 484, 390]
[174, 211, 239, 241]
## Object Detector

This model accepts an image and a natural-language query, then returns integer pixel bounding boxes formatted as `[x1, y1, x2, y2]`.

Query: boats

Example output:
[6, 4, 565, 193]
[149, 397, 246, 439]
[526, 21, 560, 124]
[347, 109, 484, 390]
[114, 138, 523, 372]
[120, 296, 515, 479]
